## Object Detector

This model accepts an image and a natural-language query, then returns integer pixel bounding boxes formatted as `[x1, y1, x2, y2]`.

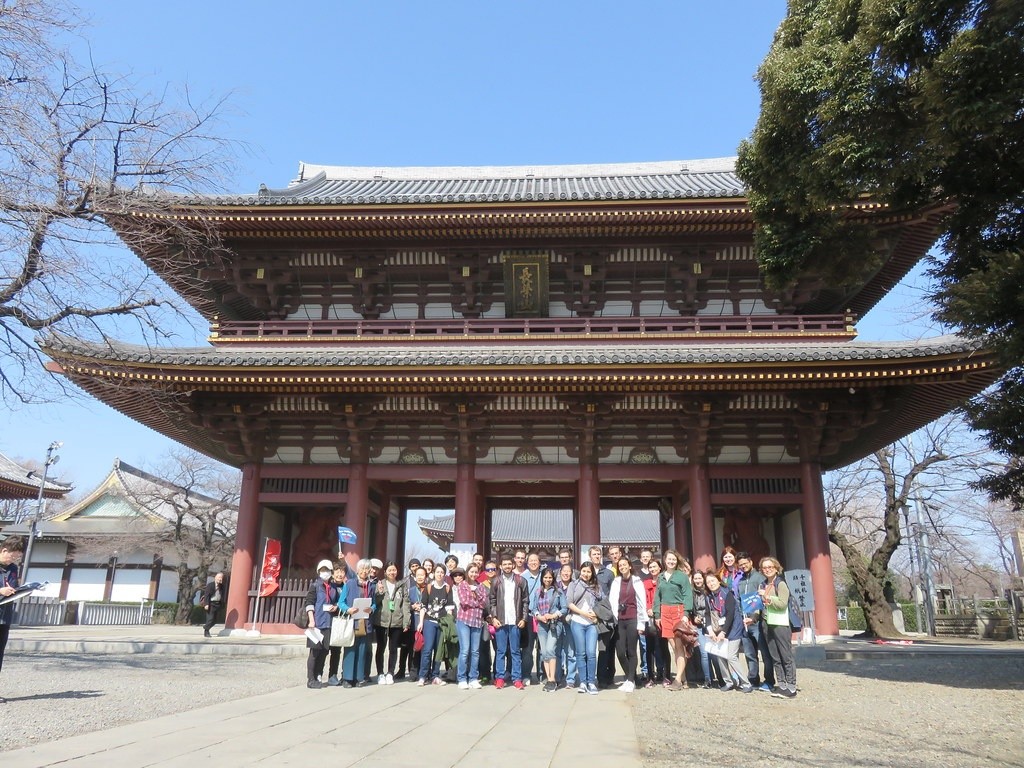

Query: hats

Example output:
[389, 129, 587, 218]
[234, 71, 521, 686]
[370, 558, 384, 569]
[316, 559, 334, 574]
[449, 568, 465, 575]
[409, 558, 421, 569]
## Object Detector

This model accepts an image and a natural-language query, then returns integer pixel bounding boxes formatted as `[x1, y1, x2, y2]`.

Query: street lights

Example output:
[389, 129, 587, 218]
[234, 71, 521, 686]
[900, 504, 923, 634]
[11, 441, 65, 624]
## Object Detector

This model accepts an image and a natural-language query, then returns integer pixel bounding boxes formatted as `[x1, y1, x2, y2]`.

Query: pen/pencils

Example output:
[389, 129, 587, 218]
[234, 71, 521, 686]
[6, 582, 15, 594]
[743, 614, 748, 632]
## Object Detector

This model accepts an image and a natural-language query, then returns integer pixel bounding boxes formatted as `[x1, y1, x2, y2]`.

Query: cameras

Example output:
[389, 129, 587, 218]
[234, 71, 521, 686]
[549, 619, 558, 632]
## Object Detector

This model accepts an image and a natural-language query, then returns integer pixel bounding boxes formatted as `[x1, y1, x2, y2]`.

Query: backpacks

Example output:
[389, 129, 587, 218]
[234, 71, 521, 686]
[774, 577, 804, 628]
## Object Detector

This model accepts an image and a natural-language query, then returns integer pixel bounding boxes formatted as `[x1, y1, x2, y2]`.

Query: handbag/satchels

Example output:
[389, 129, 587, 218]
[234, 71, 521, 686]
[293, 604, 309, 629]
[199, 595, 206, 607]
[329, 609, 355, 647]
[354, 617, 367, 637]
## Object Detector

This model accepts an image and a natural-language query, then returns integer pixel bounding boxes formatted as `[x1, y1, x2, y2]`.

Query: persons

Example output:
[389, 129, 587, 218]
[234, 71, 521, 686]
[883, 581, 895, 603]
[608, 545, 798, 699]
[0, 534, 28, 701]
[202, 571, 225, 637]
[305, 552, 489, 690]
[490, 547, 615, 694]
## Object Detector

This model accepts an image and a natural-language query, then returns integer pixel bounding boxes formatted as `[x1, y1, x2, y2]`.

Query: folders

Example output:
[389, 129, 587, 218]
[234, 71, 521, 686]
[0, 582, 41, 605]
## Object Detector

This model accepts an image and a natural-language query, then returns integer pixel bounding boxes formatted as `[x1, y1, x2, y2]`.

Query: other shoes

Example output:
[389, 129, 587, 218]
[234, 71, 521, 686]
[482, 620, 493, 643]
[302, 669, 799, 700]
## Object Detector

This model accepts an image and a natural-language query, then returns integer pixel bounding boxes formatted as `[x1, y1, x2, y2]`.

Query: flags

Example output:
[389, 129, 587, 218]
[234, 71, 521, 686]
[259, 539, 281, 597]
[338, 525, 357, 544]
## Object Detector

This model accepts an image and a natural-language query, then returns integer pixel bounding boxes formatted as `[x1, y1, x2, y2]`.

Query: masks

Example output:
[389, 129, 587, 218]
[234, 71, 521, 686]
[319, 571, 331, 580]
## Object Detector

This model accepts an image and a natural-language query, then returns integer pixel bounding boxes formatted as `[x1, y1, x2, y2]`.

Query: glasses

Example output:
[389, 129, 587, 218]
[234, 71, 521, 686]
[485, 567, 496, 572]
[453, 573, 463, 577]
[760, 566, 775, 570]
